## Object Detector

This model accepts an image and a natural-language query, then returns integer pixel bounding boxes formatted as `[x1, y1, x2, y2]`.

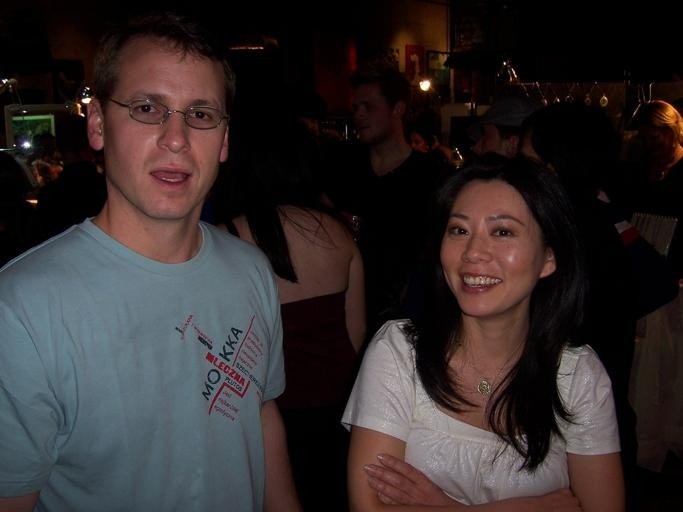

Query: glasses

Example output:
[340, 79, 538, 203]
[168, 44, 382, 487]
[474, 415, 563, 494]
[104, 96, 230, 131]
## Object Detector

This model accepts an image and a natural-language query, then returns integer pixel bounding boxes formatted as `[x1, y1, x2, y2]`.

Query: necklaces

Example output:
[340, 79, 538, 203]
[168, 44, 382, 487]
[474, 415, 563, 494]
[457, 326, 534, 397]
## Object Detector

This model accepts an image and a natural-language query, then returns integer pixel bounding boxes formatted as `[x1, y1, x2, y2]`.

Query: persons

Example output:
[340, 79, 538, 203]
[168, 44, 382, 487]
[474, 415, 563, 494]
[206, 109, 369, 511]
[2, 16, 321, 510]
[629, 212, 683, 481]
[0, 62, 683, 363]
[344, 154, 631, 511]
